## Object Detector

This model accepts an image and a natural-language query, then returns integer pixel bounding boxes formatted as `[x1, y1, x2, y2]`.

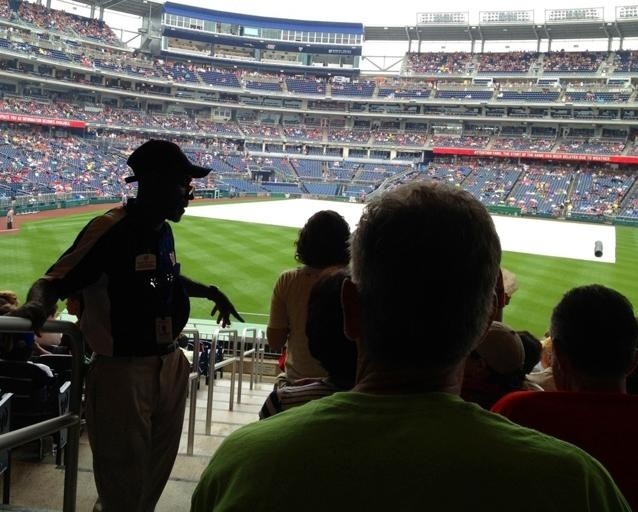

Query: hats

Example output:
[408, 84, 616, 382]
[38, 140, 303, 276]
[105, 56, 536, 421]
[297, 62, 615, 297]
[477, 320, 525, 372]
[125, 139, 213, 183]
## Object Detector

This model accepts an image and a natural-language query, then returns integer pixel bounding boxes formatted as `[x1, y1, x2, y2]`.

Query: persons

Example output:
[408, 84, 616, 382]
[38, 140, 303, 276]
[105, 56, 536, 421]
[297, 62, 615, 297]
[0, 1, 638, 221]
[188, 178, 631, 512]
[5, 207, 15, 229]
[1, 137, 246, 511]
[259, 210, 638, 512]
[0, 290, 205, 395]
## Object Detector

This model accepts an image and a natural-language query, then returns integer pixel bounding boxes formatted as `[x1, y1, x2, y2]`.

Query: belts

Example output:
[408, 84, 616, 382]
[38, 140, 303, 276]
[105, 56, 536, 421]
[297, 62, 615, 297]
[130, 345, 180, 356]
